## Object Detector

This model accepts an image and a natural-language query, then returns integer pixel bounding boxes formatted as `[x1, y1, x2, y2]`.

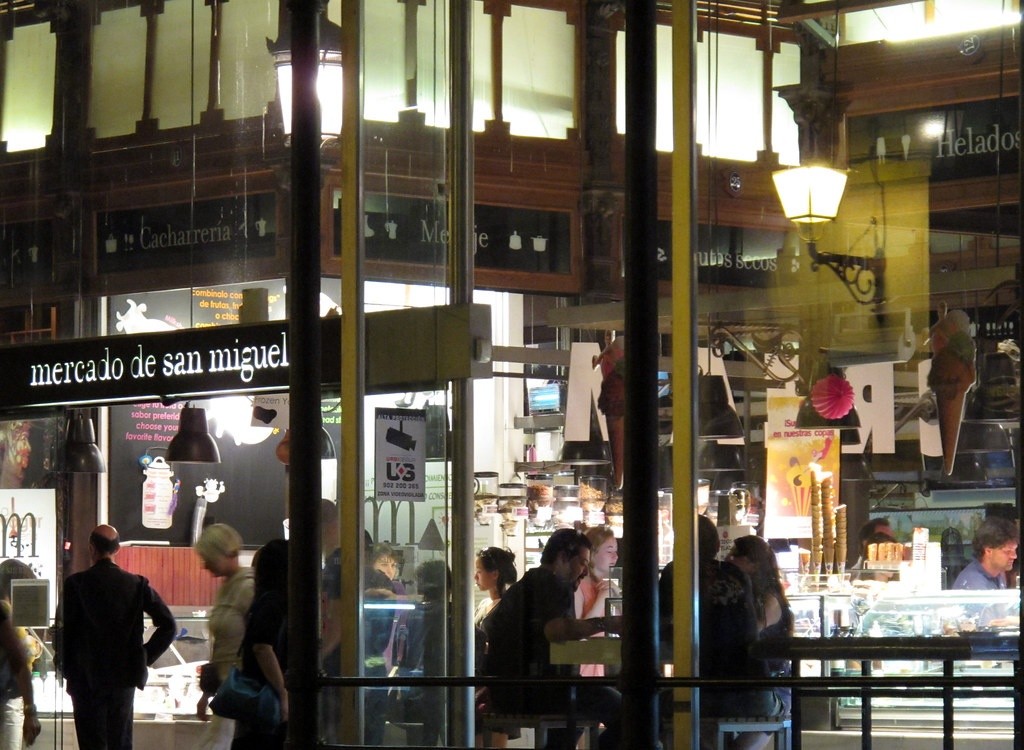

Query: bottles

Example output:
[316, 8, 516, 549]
[191, 666, 208, 712]
[473, 472, 499, 526]
[499, 483, 528, 537]
[605, 494, 624, 538]
[828, 625, 847, 677]
[870, 621, 884, 670]
[168, 669, 186, 713]
[845, 627, 862, 675]
[525, 473, 553, 527]
[31, 672, 43, 712]
[580, 476, 607, 525]
[553, 484, 581, 531]
[43, 671, 59, 712]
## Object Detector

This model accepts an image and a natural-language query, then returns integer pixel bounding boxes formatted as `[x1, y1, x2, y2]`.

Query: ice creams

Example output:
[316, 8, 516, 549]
[927, 300, 977, 475]
[596, 335, 624, 491]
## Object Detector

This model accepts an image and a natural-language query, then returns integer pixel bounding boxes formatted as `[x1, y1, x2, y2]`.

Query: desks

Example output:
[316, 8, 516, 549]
[778, 636, 1024, 750]
[550, 635, 622, 664]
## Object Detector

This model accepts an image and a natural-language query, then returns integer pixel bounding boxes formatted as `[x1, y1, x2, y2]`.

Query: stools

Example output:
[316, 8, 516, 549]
[477, 711, 598, 749]
[700, 715, 793, 750]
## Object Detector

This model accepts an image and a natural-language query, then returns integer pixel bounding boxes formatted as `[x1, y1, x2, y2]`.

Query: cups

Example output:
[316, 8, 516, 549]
[192, 612, 206, 617]
[698, 479, 710, 515]
[45, 642, 53, 661]
[706, 490, 728, 518]
[795, 618, 820, 637]
[899, 528, 942, 595]
[731, 481, 760, 514]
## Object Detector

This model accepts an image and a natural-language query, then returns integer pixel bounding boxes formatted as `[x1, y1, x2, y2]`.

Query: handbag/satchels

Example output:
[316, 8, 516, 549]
[209, 665, 281, 727]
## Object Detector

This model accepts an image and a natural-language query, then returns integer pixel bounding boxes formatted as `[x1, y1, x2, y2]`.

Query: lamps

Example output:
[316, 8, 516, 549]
[264, 11, 343, 189]
[321, 412, 336, 458]
[771, 119, 887, 318]
[556, 314, 873, 496]
[950, 0, 1021, 484]
[419, 1, 451, 464]
[54, 1, 105, 470]
[166, 0, 220, 467]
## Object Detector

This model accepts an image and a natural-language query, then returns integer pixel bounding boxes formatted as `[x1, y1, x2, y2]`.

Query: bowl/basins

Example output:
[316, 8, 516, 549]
[789, 573, 851, 594]
[885, 661, 925, 675]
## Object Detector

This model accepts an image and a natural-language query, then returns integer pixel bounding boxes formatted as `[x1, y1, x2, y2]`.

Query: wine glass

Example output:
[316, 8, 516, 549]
[729, 489, 751, 526]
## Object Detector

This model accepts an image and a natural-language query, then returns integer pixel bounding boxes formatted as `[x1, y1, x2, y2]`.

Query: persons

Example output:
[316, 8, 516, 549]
[195, 523, 255, 750]
[473, 546, 521, 741]
[51, 524, 177, 750]
[844, 518, 896, 584]
[0, 558, 42, 750]
[371, 542, 407, 675]
[234, 500, 375, 750]
[366, 558, 455, 744]
[952, 516, 1021, 630]
[850, 532, 898, 591]
[726, 534, 793, 711]
[657, 514, 788, 750]
[482, 529, 626, 750]
[574, 526, 625, 676]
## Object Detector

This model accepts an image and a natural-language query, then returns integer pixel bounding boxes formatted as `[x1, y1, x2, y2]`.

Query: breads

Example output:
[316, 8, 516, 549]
[943, 619, 976, 635]
[989, 616, 1020, 627]
